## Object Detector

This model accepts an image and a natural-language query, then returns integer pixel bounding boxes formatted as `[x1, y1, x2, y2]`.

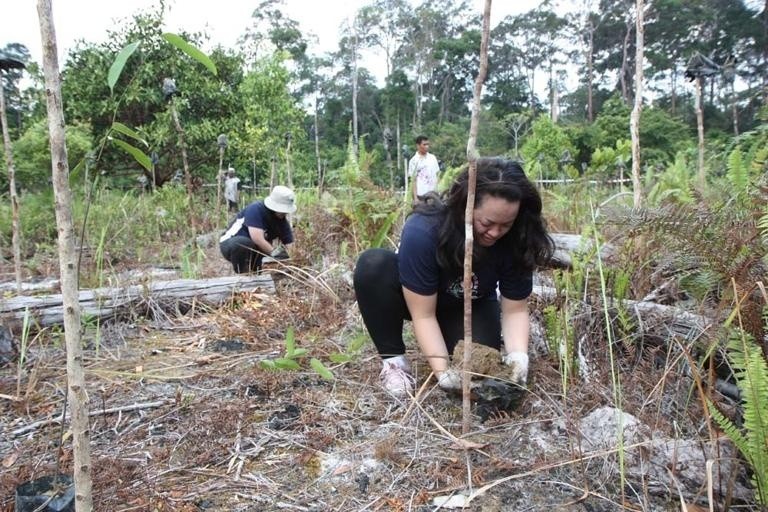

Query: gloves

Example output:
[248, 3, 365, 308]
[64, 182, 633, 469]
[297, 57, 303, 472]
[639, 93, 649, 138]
[437, 371, 476, 395]
[504, 353, 530, 389]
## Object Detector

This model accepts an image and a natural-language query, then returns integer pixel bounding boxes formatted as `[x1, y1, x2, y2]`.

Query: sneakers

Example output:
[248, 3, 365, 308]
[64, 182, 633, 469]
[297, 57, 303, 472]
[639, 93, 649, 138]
[379, 362, 416, 397]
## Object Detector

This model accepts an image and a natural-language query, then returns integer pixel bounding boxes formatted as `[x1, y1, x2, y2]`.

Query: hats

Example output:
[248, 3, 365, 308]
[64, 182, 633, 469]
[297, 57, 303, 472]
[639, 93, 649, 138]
[264, 185, 298, 214]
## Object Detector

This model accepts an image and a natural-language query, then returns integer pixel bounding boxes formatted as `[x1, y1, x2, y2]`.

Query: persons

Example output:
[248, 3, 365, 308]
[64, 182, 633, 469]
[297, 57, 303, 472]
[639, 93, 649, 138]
[407, 135, 440, 205]
[219, 185, 297, 276]
[353, 159, 556, 399]
[224, 167, 241, 213]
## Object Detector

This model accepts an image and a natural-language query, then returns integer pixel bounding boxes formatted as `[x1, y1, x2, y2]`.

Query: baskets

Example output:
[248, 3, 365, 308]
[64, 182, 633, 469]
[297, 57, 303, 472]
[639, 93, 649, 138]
[262, 258, 310, 275]
